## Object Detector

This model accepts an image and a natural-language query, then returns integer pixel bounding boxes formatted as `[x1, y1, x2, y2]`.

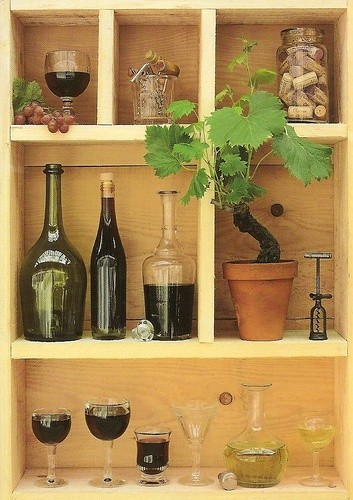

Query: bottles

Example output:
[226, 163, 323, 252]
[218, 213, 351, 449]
[142, 191, 196, 340]
[223, 382, 289, 488]
[218, 471, 238, 491]
[276, 26, 330, 123]
[20, 164, 87, 342]
[144, 49, 180, 76]
[91, 172, 126, 340]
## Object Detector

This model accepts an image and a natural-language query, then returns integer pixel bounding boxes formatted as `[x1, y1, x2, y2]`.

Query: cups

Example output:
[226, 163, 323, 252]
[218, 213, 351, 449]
[130, 74, 178, 124]
[133, 424, 172, 487]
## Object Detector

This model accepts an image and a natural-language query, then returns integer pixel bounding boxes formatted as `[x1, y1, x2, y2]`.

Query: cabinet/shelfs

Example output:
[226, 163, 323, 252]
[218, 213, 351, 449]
[1, 0, 353, 496]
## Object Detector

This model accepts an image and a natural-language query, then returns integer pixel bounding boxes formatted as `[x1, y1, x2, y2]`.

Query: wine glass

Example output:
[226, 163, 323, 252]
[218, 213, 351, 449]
[171, 398, 218, 486]
[44, 50, 91, 116]
[32, 407, 71, 488]
[297, 409, 336, 487]
[85, 397, 130, 488]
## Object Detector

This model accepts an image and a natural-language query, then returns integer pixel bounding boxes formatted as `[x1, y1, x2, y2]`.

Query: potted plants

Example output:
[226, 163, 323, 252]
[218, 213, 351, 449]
[144, 34, 334, 340]
